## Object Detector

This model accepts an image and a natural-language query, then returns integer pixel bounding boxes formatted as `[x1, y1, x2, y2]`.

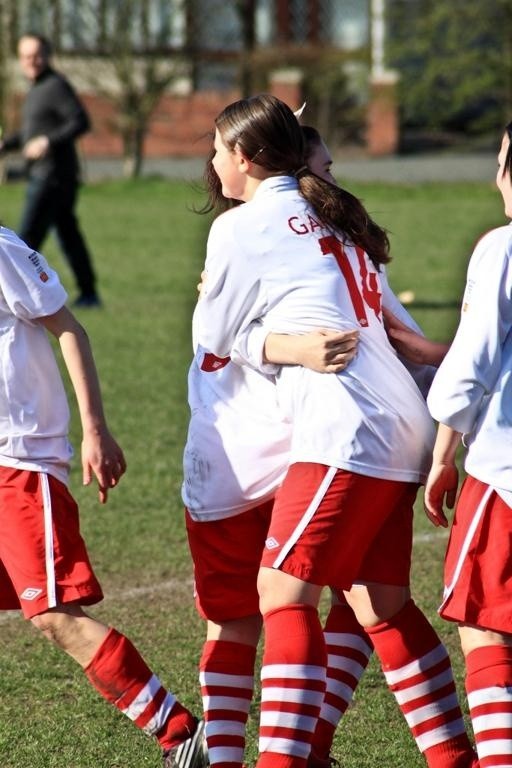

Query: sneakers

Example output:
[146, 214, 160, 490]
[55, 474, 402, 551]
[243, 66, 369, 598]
[162, 719, 208, 768]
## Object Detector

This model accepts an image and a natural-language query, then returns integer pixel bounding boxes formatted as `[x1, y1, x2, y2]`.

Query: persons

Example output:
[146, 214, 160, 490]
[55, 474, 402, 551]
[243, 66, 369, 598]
[0, 225, 212, 766]
[0, 33, 101, 306]
[424, 123, 512, 767]
